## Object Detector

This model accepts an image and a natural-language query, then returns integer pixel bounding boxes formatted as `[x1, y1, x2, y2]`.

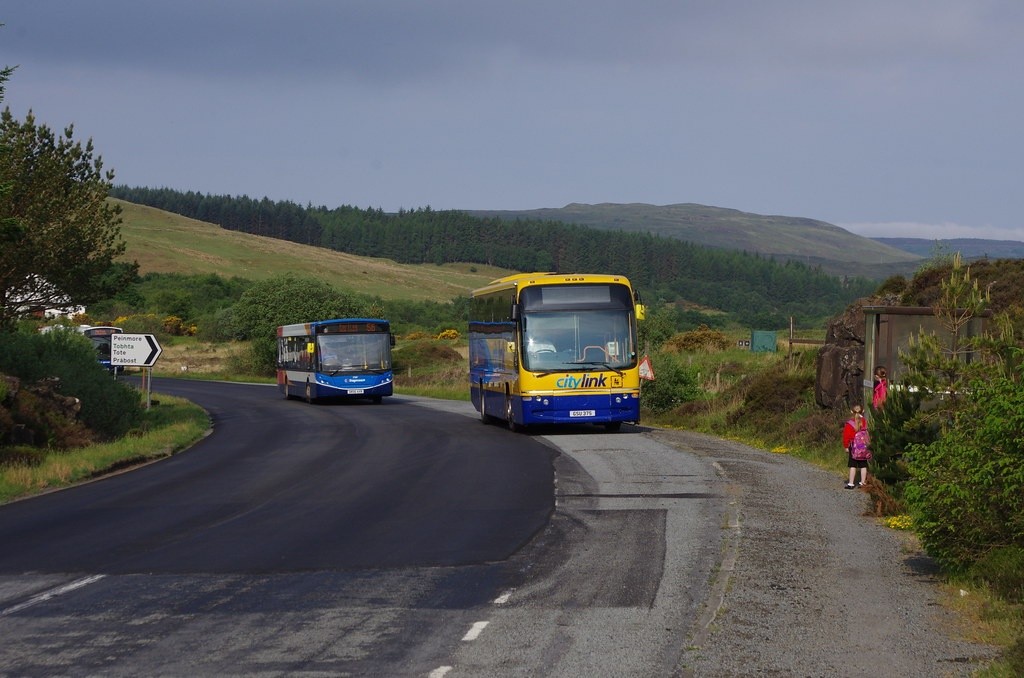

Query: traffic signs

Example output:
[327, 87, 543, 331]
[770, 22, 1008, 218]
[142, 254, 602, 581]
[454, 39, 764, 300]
[112, 332, 163, 366]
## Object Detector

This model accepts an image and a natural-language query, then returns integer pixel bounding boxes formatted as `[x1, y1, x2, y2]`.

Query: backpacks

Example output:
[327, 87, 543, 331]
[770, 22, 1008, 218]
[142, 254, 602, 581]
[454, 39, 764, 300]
[846, 419, 872, 460]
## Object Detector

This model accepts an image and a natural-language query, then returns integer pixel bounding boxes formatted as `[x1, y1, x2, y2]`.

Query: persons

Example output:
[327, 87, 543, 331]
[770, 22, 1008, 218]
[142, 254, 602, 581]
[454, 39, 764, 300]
[842, 404, 868, 489]
[872, 364, 889, 411]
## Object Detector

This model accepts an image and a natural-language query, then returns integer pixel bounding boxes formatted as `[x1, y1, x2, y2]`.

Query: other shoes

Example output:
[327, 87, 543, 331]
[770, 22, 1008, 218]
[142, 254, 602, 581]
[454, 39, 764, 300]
[858, 482, 866, 486]
[844, 484, 855, 489]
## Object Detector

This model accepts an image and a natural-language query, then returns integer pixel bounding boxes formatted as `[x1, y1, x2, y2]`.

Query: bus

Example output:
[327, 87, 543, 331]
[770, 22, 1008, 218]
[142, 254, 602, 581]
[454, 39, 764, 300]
[276, 318, 396, 405]
[467, 270, 645, 433]
[81, 326, 125, 373]
